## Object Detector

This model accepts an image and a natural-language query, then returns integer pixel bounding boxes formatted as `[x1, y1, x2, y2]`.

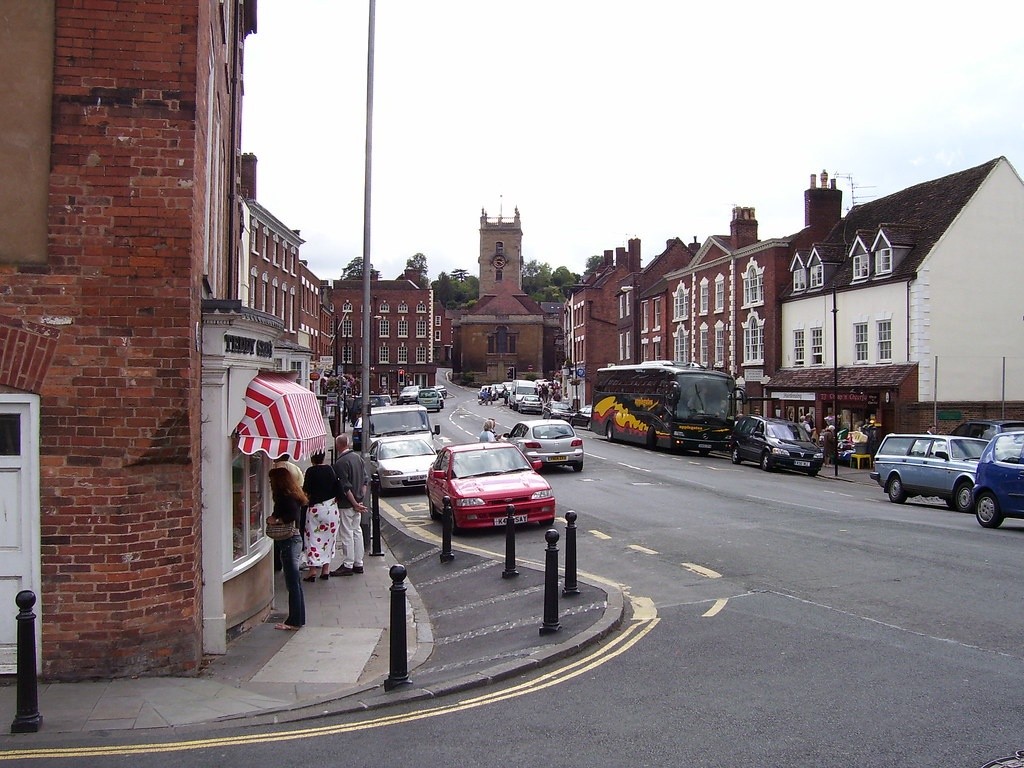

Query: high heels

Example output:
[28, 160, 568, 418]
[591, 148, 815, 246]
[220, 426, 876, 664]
[303, 575, 316, 582]
[319, 573, 329, 579]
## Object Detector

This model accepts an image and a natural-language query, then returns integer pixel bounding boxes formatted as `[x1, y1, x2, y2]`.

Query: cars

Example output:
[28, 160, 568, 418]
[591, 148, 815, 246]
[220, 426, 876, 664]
[367, 434, 440, 497]
[424, 441, 556, 535]
[342, 384, 448, 451]
[478, 377, 593, 432]
[947, 420, 1024, 442]
[970, 429, 1024, 529]
[496, 419, 584, 473]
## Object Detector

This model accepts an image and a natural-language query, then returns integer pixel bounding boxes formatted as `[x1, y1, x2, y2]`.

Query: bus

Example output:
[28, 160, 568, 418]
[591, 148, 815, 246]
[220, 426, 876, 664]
[590, 358, 748, 458]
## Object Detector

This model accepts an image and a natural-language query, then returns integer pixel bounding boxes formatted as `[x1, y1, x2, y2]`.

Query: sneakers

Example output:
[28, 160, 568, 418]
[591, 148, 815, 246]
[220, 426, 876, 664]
[330, 564, 352, 576]
[353, 565, 364, 573]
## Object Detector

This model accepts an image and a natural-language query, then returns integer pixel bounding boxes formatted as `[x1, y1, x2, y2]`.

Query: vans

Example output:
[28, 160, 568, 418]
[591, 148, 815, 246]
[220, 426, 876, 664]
[869, 433, 992, 513]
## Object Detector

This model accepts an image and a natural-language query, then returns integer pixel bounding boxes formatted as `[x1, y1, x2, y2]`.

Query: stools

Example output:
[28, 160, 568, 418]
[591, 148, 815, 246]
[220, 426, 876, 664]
[850, 454, 871, 471]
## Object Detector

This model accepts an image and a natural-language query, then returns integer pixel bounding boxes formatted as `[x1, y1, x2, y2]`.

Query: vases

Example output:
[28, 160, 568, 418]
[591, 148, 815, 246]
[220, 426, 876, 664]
[855, 443, 867, 454]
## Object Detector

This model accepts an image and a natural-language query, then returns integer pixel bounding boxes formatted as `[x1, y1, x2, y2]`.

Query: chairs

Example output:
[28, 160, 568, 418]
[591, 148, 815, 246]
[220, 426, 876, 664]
[971, 429, 981, 438]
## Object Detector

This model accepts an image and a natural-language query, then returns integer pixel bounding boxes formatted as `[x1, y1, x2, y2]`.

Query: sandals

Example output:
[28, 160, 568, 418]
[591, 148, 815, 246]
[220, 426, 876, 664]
[274, 621, 299, 630]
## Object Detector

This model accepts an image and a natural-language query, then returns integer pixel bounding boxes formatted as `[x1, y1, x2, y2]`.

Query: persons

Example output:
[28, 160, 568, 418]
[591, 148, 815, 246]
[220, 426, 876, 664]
[538, 382, 561, 403]
[353, 412, 362, 428]
[502, 386, 508, 406]
[329, 435, 369, 577]
[799, 412, 816, 440]
[823, 425, 835, 468]
[926, 425, 936, 435]
[483, 387, 493, 406]
[266, 454, 309, 631]
[302, 450, 341, 583]
[479, 418, 502, 442]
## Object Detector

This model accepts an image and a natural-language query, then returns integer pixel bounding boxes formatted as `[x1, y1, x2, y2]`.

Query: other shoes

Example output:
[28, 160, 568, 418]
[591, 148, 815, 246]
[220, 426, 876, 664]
[825, 465, 832, 467]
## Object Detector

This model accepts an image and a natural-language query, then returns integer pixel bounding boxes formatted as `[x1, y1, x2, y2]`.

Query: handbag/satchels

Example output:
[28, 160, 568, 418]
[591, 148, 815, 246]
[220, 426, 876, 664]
[266, 513, 295, 540]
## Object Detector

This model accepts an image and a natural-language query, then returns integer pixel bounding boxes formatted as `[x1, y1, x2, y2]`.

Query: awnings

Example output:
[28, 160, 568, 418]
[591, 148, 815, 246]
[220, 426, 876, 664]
[236, 374, 327, 461]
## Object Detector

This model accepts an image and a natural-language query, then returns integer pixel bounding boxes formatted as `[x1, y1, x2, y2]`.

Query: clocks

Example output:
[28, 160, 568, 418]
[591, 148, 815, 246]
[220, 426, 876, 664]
[492, 254, 506, 269]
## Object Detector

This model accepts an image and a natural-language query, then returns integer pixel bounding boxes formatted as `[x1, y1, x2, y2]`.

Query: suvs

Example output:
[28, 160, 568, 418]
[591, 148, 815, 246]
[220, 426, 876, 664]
[727, 414, 824, 477]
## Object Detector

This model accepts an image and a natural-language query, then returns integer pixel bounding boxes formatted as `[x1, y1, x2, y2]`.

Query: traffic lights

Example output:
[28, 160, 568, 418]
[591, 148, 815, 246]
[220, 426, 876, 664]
[506, 367, 515, 379]
[398, 370, 404, 384]
